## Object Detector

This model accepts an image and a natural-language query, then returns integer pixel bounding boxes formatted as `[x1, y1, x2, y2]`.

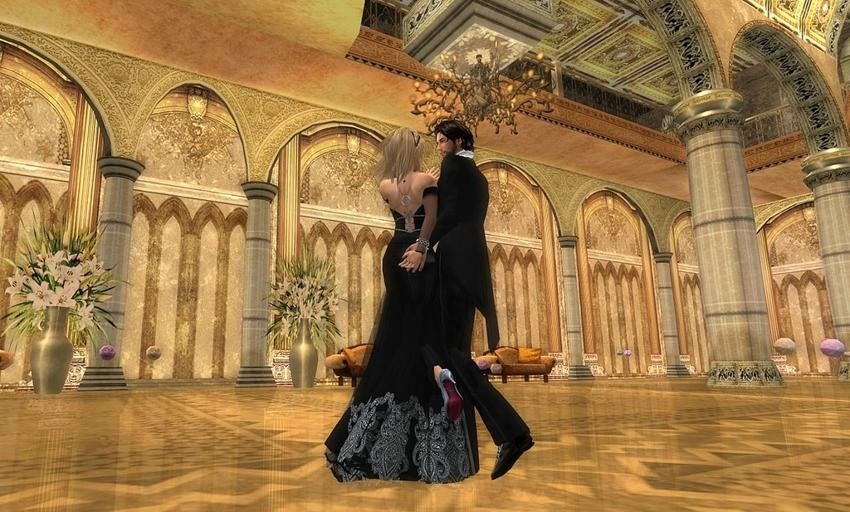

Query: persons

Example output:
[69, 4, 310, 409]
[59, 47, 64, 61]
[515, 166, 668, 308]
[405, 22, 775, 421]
[403, 118, 535, 481]
[320, 124, 482, 485]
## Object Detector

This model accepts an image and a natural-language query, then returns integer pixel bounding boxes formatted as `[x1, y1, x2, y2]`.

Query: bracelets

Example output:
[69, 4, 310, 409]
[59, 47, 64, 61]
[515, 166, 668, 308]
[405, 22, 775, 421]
[415, 239, 429, 255]
